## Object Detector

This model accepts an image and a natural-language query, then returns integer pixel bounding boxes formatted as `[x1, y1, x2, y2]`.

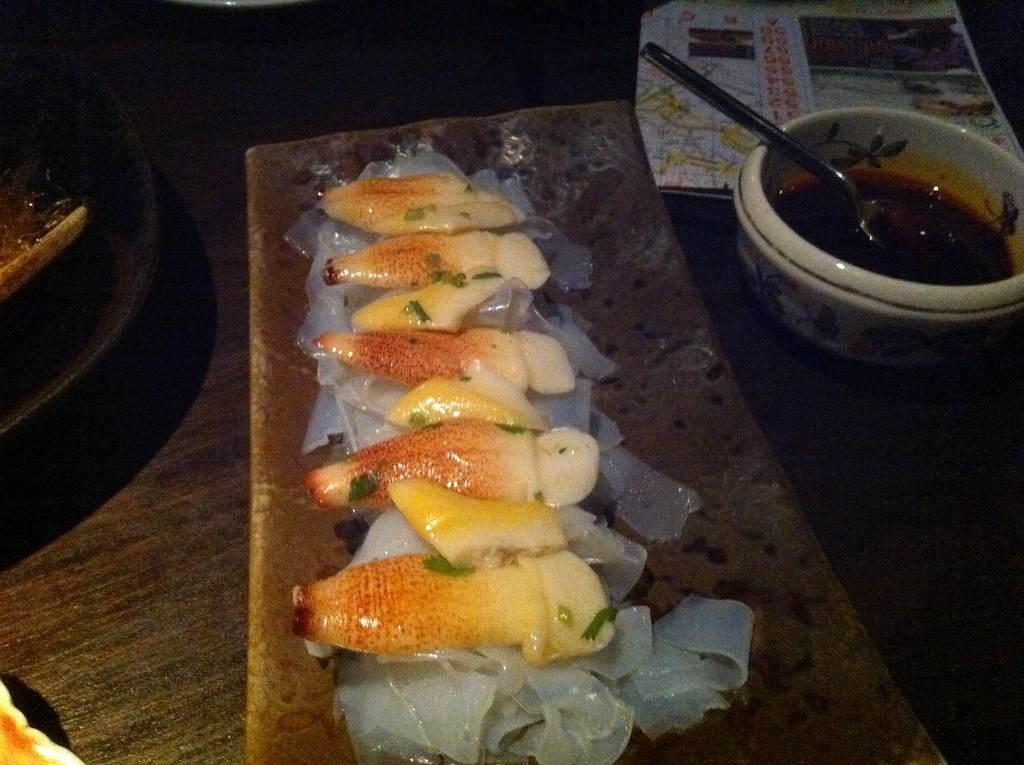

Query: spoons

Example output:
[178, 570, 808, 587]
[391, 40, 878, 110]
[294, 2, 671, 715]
[639, 41, 938, 256]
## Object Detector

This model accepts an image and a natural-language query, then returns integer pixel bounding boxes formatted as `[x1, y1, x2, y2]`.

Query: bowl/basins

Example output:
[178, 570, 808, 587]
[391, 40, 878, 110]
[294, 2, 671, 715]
[735, 106, 1024, 368]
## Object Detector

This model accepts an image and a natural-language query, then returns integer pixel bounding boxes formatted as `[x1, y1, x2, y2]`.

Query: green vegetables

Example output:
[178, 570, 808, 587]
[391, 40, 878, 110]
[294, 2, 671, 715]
[345, 206, 618, 643]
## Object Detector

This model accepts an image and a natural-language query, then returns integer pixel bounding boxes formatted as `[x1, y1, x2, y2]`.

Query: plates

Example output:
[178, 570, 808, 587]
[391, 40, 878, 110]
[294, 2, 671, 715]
[0, 51, 162, 433]
[244, 101, 951, 764]
